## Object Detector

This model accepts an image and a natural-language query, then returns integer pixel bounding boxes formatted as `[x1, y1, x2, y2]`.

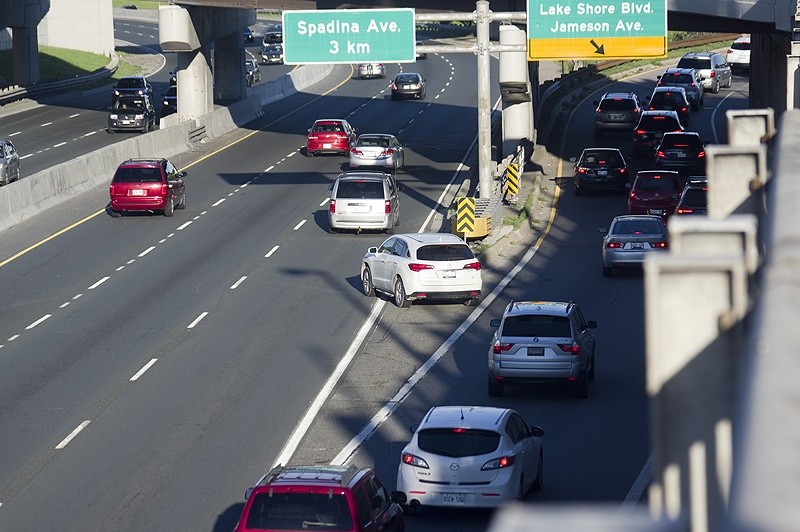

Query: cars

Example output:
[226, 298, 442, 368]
[0, 139, 21, 186]
[167, 65, 177, 86]
[160, 85, 177, 115]
[394, 405, 545, 512]
[245, 21, 284, 88]
[121, 5, 137, 10]
[306, 117, 358, 156]
[356, 41, 428, 101]
[655, 33, 751, 113]
[600, 214, 672, 280]
[111, 76, 153, 102]
[348, 133, 406, 174]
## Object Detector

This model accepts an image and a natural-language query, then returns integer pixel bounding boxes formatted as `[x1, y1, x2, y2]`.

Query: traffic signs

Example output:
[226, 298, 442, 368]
[527, 0, 668, 59]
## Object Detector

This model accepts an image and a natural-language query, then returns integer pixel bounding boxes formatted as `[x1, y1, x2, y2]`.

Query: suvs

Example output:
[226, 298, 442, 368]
[592, 91, 641, 133]
[105, 94, 156, 134]
[652, 130, 712, 175]
[233, 461, 408, 532]
[360, 232, 482, 308]
[625, 168, 688, 219]
[645, 87, 695, 128]
[110, 158, 188, 219]
[569, 147, 631, 195]
[672, 175, 707, 216]
[487, 297, 600, 401]
[328, 170, 401, 236]
[632, 110, 688, 157]
